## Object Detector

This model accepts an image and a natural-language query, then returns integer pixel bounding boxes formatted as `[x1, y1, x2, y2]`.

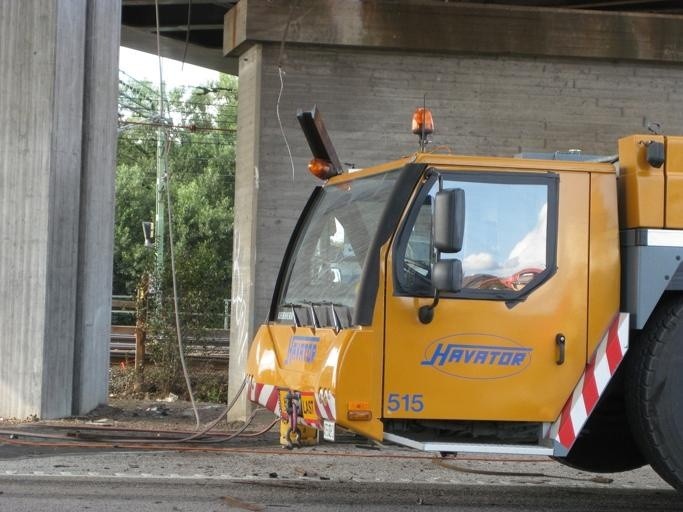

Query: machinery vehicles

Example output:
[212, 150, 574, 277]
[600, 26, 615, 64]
[242, 94, 682, 499]
